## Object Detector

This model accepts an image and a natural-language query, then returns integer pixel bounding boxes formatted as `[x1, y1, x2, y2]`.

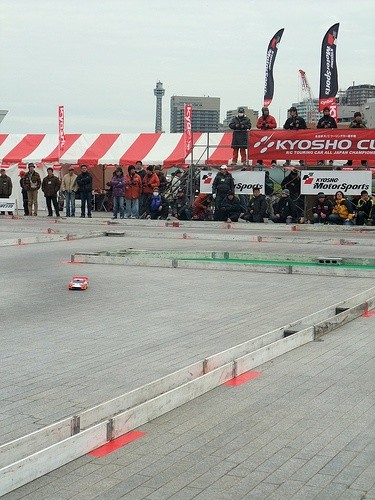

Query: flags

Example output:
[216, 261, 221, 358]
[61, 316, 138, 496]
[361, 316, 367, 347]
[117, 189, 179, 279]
[59, 106, 65, 151]
[319, 23, 340, 124]
[264, 28, 284, 108]
[185, 104, 193, 153]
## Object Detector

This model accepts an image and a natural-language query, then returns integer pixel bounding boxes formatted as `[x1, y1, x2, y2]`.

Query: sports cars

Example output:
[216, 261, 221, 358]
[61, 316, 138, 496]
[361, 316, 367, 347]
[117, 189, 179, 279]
[68, 276, 88, 290]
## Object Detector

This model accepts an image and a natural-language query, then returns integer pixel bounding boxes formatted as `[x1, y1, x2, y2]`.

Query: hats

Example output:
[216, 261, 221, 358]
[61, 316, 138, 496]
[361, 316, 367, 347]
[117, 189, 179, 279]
[318, 192, 325, 199]
[128, 165, 135, 172]
[220, 165, 227, 169]
[288, 107, 297, 112]
[28, 163, 35, 168]
[115, 167, 121, 172]
[238, 108, 245, 113]
[68, 168, 74, 171]
[18, 170, 25, 176]
[281, 189, 289, 196]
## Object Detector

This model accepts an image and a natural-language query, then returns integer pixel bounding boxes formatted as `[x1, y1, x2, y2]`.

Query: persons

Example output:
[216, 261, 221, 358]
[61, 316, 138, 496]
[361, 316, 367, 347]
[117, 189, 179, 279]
[316, 107, 338, 165]
[77, 165, 93, 218]
[256, 107, 276, 166]
[283, 107, 307, 166]
[342, 112, 368, 166]
[106, 159, 375, 226]
[229, 107, 251, 165]
[0, 163, 61, 216]
[62, 168, 78, 217]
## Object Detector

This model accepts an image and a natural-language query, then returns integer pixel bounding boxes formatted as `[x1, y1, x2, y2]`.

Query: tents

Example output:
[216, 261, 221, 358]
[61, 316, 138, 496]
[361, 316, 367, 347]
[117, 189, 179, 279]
[0, 132, 375, 173]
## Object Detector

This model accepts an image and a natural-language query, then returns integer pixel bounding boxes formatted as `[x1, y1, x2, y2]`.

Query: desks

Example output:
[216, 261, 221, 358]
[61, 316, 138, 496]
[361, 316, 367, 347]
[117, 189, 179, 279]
[93, 193, 107, 212]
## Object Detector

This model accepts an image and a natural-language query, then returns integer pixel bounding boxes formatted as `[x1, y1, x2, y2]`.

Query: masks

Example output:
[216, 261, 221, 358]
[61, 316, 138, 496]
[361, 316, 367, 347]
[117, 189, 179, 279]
[153, 191, 159, 196]
[239, 113, 243, 116]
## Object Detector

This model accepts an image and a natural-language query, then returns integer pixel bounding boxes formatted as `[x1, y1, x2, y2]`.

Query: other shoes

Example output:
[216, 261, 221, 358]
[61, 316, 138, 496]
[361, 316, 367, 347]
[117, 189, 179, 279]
[231, 162, 237, 165]
[363, 162, 368, 167]
[329, 161, 333, 166]
[318, 162, 324, 166]
[300, 162, 304, 165]
[283, 162, 291, 166]
[344, 162, 351, 166]
[272, 163, 276, 166]
[243, 162, 246, 165]
[256, 163, 263, 166]
[79, 214, 168, 220]
